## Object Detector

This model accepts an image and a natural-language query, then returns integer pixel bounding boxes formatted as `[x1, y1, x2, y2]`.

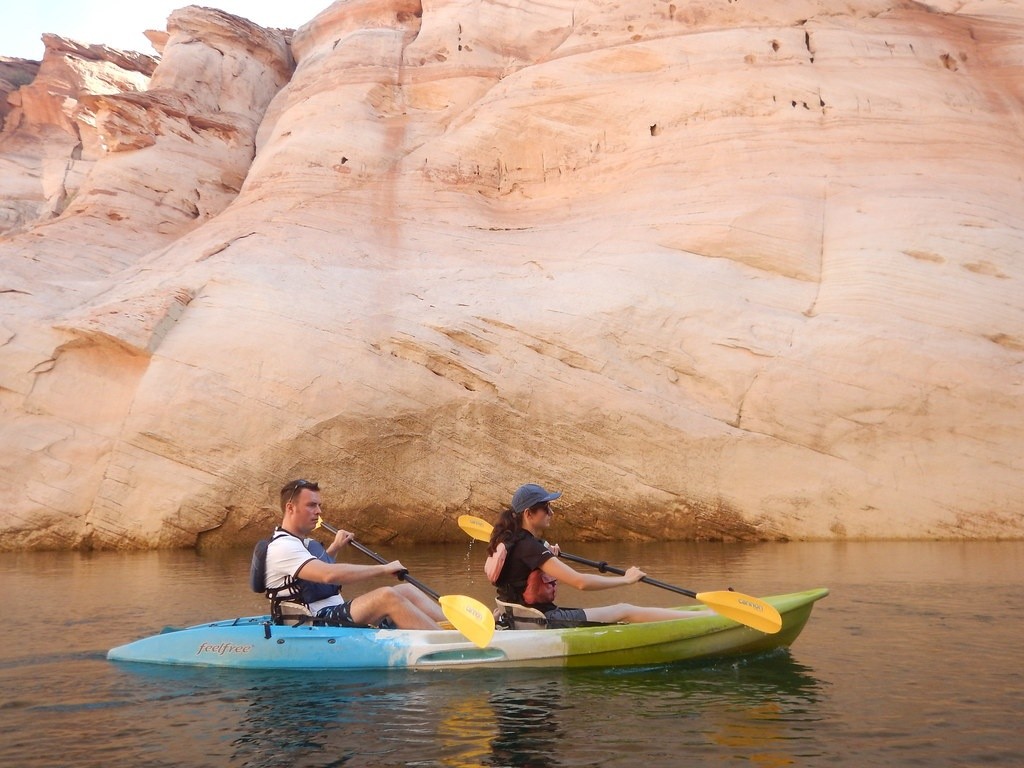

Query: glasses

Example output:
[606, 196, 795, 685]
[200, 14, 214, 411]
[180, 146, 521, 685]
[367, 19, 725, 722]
[540, 502, 551, 510]
[289, 479, 307, 502]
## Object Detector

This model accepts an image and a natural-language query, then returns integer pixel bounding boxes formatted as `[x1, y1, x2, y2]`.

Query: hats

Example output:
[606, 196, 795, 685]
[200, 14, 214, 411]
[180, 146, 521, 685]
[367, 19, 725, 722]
[512, 484, 561, 514]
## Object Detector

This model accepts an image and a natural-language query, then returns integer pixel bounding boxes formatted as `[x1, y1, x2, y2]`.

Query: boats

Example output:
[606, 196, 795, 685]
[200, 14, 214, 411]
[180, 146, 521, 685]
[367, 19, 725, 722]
[102, 585, 832, 683]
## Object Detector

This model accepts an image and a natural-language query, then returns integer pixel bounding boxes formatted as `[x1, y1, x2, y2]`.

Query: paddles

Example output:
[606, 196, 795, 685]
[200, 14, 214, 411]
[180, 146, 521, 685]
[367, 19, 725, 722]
[457, 513, 784, 636]
[309, 513, 497, 650]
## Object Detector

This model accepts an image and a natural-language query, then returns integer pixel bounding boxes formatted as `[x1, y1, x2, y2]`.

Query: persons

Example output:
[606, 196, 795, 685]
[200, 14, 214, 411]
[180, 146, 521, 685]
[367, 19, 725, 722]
[487, 484, 717, 628]
[264, 479, 448, 630]
